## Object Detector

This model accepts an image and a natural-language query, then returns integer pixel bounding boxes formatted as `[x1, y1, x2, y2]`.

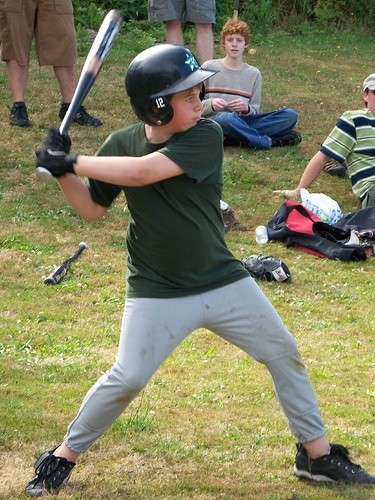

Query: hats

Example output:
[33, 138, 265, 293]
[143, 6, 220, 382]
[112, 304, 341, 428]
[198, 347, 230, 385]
[362, 73, 375, 92]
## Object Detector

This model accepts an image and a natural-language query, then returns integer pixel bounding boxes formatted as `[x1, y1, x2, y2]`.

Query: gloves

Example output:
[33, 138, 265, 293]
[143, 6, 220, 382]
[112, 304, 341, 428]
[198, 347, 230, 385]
[42, 125, 72, 155]
[35, 146, 79, 178]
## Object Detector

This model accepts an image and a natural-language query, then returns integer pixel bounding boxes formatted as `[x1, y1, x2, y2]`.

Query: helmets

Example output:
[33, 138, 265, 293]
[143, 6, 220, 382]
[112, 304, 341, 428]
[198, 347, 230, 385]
[124, 44, 221, 100]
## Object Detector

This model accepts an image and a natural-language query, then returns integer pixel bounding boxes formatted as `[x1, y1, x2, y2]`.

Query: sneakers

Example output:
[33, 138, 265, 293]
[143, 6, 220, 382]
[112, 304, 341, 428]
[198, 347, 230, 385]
[25, 444, 77, 497]
[293, 442, 375, 485]
[58, 102, 104, 128]
[9, 101, 30, 126]
[271, 130, 303, 147]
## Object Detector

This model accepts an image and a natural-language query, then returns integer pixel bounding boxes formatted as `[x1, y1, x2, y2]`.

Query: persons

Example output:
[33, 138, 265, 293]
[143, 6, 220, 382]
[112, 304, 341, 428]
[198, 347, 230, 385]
[146, 0, 216, 65]
[0, 0, 104, 129]
[199, 20, 302, 149]
[272, 73, 375, 210]
[24, 43, 375, 499]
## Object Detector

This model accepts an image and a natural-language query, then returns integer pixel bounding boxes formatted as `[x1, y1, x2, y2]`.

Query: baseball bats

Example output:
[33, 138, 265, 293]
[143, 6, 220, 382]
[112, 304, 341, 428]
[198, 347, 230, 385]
[44, 242, 87, 284]
[36, 9, 123, 180]
